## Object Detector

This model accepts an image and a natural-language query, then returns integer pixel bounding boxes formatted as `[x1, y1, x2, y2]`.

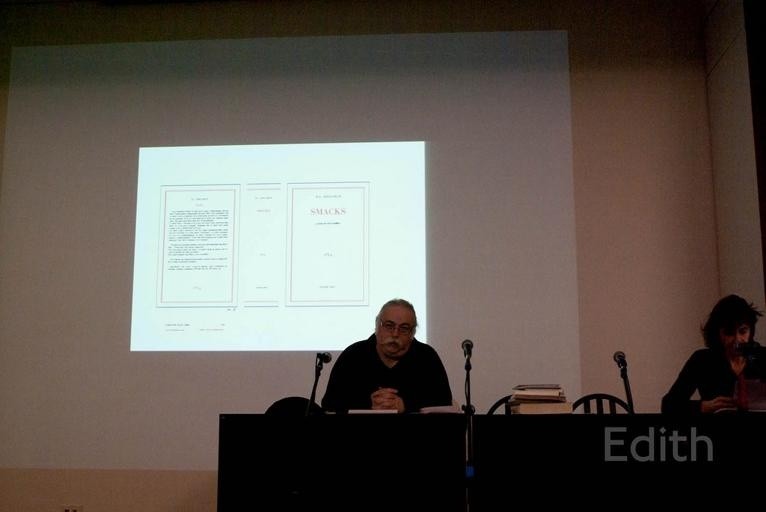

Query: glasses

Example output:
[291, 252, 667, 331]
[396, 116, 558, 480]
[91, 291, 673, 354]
[378, 319, 415, 335]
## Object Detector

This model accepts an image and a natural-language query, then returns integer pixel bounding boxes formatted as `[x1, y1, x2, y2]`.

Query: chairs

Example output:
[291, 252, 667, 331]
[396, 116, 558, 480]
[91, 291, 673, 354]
[486, 394, 512, 415]
[574, 394, 629, 414]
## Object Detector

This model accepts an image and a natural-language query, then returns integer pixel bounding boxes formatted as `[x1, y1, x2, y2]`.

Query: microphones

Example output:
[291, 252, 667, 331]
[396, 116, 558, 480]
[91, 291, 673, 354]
[317, 352, 331, 363]
[462, 340, 473, 360]
[614, 351, 626, 369]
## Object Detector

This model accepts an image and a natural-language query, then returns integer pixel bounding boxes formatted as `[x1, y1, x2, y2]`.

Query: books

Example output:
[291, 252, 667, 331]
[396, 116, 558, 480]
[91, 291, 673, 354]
[508, 384, 573, 414]
[348, 409, 397, 414]
[420, 406, 456, 414]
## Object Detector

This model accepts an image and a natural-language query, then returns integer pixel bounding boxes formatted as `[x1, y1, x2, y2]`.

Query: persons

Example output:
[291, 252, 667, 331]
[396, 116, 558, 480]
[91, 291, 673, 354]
[661, 295, 765, 416]
[321, 298, 453, 412]
